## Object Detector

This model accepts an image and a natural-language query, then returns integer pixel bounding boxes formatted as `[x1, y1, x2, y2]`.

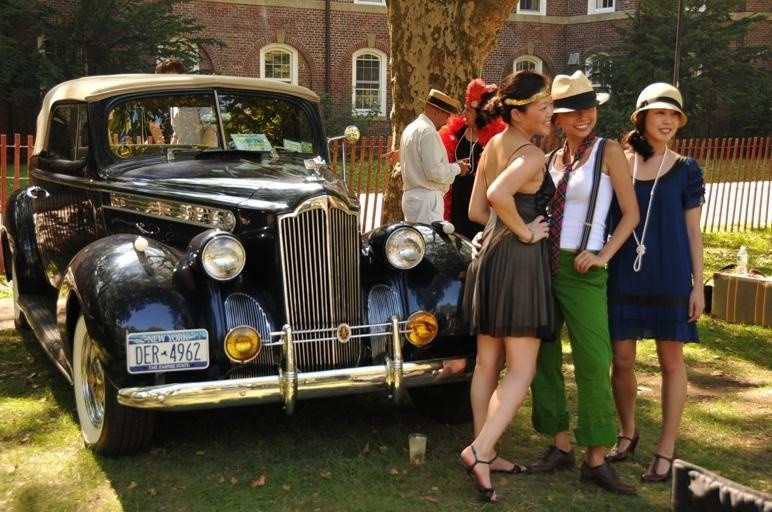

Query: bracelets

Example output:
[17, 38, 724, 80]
[519, 222, 536, 243]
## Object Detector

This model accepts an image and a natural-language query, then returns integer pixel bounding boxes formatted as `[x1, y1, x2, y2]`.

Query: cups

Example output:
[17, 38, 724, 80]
[408, 432, 428, 466]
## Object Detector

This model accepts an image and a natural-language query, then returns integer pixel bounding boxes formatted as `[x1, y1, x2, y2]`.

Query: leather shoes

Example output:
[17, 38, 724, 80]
[526, 446, 576, 473]
[579, 462, 637, 496]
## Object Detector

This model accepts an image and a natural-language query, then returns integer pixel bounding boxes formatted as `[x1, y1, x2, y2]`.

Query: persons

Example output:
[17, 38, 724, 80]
[400, 89, 471, 232]
[437, 77, 508, 223]
[603, 82, 707, 485]
[461, 70, 557, 502]
[469, 69, 644, 493]
[148, 56, 193, 145]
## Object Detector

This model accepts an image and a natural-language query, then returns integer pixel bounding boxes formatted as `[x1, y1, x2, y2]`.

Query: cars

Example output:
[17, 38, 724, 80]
[0, 72, 486, 460]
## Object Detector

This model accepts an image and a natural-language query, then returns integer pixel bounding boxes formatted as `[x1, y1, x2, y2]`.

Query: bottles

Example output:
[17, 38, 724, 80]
[735, 245, 748, 276]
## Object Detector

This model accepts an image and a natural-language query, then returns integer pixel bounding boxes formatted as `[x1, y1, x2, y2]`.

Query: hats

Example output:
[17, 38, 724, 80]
[630, 82, 688, 128]
[551, 70, 609, 113]
[419, 89, 459, 114]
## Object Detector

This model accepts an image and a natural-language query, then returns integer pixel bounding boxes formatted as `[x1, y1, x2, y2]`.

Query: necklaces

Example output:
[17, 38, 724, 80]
[628, 145, 671, 272]
[455, 126, 480, 175]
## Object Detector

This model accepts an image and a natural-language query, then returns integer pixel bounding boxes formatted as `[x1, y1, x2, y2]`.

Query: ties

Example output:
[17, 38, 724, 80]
[550, 135, 596, 275]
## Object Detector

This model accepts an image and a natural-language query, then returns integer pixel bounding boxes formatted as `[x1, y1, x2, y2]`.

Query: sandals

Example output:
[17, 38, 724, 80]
[459, 445, 526, 503]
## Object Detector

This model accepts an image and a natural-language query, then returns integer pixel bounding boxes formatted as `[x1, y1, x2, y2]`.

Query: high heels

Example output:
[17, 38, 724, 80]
[641, 455, 676, 482]
[605, 430, 639, 462]
